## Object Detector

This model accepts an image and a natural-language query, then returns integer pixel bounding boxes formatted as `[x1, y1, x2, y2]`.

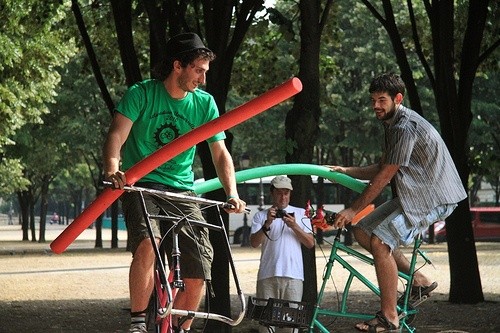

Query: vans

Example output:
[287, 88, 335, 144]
[422, 206, 500, 243]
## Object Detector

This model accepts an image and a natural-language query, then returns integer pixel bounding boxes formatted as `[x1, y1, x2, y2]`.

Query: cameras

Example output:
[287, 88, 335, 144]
[273, 209, 286, 218]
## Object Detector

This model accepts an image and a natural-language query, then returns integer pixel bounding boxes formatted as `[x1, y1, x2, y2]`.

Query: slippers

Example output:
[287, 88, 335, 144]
[355, 310, 401, 333]
[400, 280, 439, 310]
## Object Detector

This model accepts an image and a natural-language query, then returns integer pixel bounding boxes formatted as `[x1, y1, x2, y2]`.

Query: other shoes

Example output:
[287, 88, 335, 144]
[128, 322, 147, 333]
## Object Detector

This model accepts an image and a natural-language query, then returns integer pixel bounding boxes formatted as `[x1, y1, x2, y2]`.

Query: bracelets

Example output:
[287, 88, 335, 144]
[227, 196, 237, 199]
[265, 227, 270, 231]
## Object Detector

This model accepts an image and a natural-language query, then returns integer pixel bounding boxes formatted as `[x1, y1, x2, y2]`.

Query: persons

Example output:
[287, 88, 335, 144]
[249, 175, 314, 333]
[7, 207, 14, 224]
[102, 33, 246, 333]
[328, 76, 468, 333]
[52, 213, 58, 223]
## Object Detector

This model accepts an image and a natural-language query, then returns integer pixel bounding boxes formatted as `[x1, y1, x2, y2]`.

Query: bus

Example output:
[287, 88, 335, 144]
[193, 165, 391, 245]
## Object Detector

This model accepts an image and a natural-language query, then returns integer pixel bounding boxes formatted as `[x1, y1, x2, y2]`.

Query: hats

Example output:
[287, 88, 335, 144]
[165, 31, 206, 60]
[269, 175, 294, 191]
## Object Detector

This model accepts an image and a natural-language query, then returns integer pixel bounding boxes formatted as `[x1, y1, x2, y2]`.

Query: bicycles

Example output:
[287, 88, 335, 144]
[97, 180, 436, 333]
